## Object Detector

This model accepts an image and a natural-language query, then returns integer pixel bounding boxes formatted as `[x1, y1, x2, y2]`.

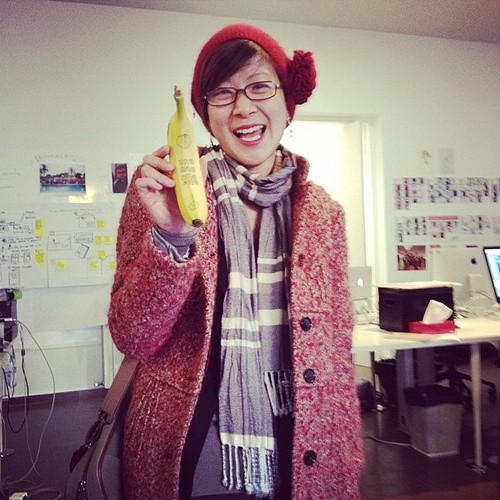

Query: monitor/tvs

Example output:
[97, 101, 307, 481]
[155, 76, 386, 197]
[429, 247, 496, 317]
[346, 266, 372, 300]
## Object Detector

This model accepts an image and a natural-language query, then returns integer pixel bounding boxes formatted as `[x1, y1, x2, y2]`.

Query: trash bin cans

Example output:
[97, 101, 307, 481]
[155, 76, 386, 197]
[402, 384, 465, 457]
[372, 357, 397, 408]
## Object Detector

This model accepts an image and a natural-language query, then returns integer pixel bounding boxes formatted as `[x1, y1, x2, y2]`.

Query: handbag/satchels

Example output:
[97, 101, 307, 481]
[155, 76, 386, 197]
[61, 354, 139, 500]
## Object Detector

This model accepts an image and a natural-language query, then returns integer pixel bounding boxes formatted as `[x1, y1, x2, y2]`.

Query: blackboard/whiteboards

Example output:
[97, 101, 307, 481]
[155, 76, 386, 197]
[0, 150, 146, 290]
[384, 175, 500, 284]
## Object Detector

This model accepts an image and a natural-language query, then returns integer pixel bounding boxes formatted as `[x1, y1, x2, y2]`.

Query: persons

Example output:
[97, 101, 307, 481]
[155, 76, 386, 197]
[108, 24, 365, 500]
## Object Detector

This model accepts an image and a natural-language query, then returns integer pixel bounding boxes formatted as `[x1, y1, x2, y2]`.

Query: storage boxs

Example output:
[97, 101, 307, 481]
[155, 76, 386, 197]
[379, 287, 454, 331]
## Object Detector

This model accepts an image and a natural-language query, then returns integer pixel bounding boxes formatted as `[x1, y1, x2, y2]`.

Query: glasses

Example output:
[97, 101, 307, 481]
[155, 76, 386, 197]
[201, 80, 283, 107]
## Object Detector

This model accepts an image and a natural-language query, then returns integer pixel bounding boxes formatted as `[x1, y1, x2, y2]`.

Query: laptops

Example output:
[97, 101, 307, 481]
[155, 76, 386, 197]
[483, 245, 500, 304]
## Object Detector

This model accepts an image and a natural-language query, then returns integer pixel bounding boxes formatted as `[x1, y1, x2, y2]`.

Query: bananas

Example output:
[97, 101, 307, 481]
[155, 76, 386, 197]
[166, 87, 208, 227]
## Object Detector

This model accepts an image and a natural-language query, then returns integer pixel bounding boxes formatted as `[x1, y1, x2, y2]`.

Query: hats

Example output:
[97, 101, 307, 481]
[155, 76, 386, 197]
[189, 23, 319, 136]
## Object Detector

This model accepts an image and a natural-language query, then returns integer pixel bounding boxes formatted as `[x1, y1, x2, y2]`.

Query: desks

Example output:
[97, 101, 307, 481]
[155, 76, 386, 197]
[350, 314, 500, 471]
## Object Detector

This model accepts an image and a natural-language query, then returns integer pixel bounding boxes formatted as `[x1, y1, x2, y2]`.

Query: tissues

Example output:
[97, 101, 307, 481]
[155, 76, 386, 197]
[409, 300, 455, 334]
